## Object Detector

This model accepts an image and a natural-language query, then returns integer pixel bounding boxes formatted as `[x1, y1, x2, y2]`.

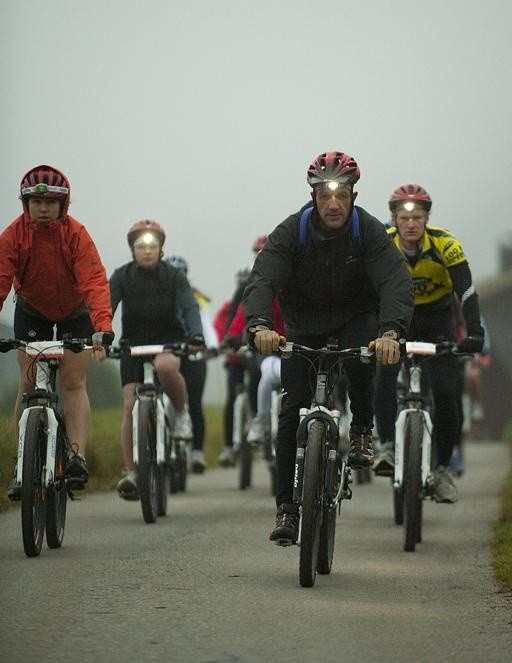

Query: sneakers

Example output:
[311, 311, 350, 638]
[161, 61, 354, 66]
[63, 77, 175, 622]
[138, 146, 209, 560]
[246, 416, 263, 443]
[7, 464, 22, 501]
[270, 503, 300, 541]
[118, 469, 139, 501]
[348, 425, 374, 466]
[218, 446, 235, 468]
[190, 449, 205, 472]
[372, 441, 395, 470]
[170, 410, 193, 440]
[65, 453, 89, 490]
[431, 465, 457, 503]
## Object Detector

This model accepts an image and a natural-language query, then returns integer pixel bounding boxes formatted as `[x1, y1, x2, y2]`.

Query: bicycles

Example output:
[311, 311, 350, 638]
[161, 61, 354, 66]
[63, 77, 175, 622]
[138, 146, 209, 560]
[210, 345, 259, 489]
[1, 331, 114, 558]
[253, 333, 411, 589]
[353, 464, 372, 485]
[373, 333, 478, 554]
[102, 338, 207, 525]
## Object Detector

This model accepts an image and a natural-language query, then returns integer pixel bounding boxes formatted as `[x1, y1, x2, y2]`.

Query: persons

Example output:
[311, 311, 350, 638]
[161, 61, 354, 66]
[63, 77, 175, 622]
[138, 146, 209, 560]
[93, 219, 206, 500]
[0, 164, 115, 501]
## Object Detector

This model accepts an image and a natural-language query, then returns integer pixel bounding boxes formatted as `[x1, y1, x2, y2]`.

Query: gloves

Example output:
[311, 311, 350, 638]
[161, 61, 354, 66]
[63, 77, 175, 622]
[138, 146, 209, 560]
[456, 335, 484, 352]
[253, 325, 286, 355]
[368, 329, 401, 365]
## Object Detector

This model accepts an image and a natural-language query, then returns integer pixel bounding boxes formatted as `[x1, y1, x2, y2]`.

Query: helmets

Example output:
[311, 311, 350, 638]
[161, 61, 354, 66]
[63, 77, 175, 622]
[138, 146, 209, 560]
[127, 220, 165, 245]
[19, 168, 69, 200]
[164, 255, 188, 275]
[389, 183, 432, 211]
[307, 150, 360, 185]
